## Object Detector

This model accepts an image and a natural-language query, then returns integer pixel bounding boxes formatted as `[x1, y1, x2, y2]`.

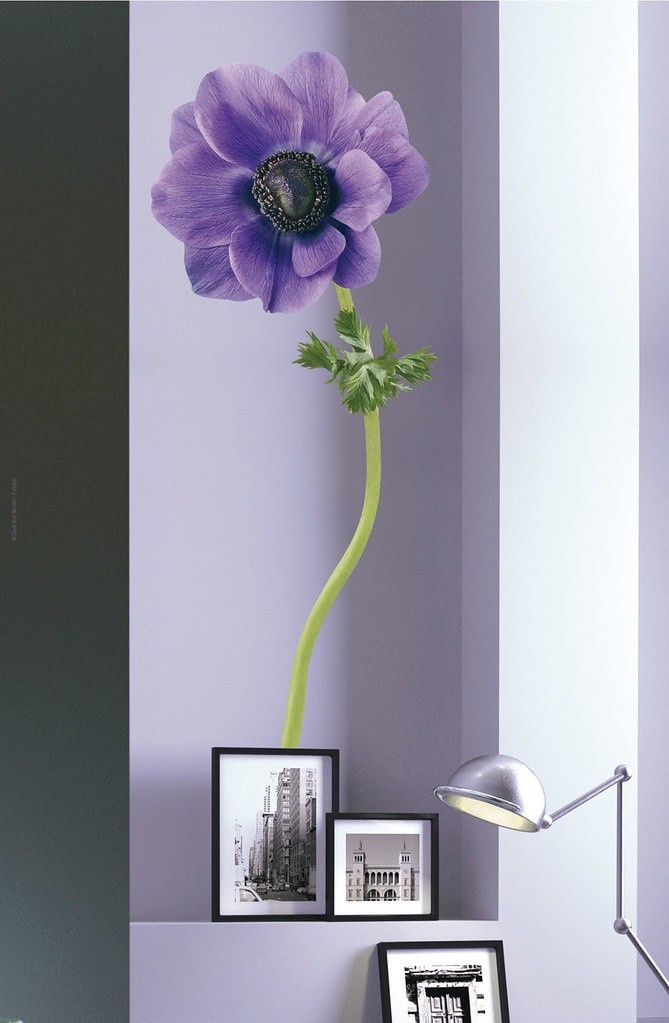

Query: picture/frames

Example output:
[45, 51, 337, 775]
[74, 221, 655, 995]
[207, 743, 344, 923]
[375, 936, 514, 1023]
[323, 810, 441, 924]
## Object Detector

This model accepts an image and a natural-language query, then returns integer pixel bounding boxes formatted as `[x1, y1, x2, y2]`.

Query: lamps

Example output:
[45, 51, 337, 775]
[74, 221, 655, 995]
[435, 748, 669, 995]
